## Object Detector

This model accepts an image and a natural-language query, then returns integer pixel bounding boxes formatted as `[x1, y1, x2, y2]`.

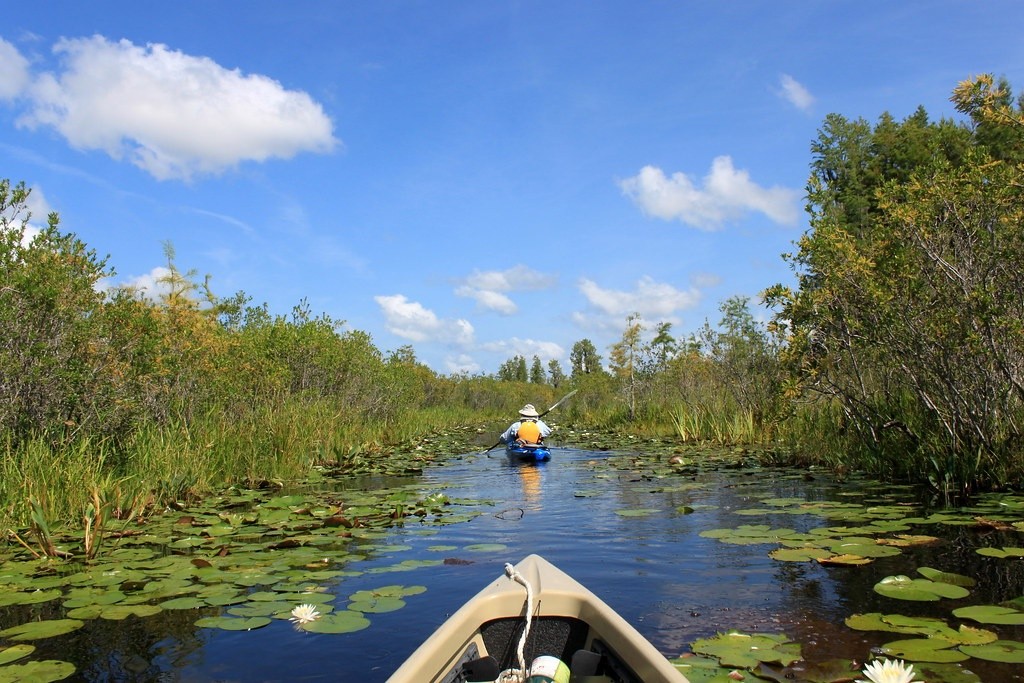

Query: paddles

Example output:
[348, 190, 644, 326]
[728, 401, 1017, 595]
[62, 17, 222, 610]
[478, 386, 580, 458]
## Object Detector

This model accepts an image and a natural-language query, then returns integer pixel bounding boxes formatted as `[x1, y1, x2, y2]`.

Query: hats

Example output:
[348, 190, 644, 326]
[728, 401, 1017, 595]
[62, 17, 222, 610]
[518, 404, 538, 416]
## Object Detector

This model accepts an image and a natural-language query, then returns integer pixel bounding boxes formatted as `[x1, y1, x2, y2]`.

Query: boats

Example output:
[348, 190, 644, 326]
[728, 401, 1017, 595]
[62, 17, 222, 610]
[507, 441, 552, 464]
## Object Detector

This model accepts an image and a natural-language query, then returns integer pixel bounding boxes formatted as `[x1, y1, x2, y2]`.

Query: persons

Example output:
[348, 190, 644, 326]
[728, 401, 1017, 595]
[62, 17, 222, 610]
[499, 404, 552, 445]
